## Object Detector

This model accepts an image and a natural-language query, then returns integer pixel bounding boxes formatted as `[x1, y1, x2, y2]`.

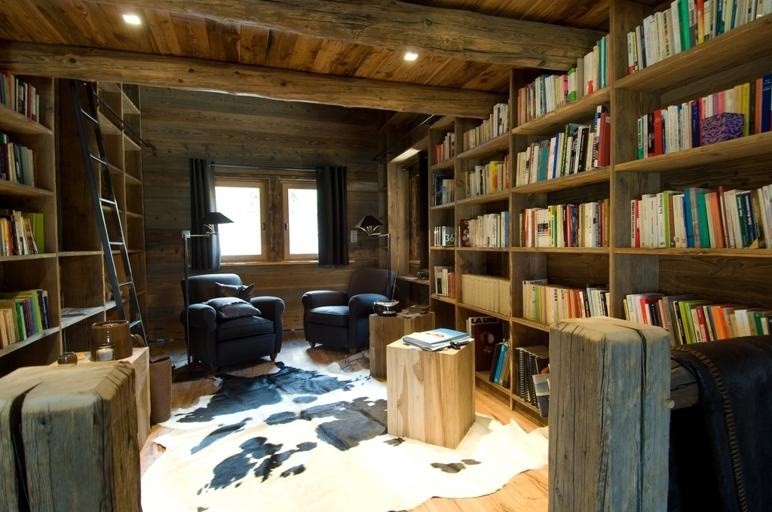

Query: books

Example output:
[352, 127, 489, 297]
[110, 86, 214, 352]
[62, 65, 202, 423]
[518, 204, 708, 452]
[462, 101, 510, 388]
[432, 135, 457, 298]
[402, 327, 470, 353]
[515, 344, 548, 418]
[0, 67, 51, 350]
[623, 1, 772, 349]
[516, 34, 610, 327]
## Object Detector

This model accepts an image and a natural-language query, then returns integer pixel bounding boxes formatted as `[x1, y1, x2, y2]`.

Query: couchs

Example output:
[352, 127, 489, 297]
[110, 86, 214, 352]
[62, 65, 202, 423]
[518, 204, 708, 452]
[300, 264, 401, 356]
[178, 272, 288, 376]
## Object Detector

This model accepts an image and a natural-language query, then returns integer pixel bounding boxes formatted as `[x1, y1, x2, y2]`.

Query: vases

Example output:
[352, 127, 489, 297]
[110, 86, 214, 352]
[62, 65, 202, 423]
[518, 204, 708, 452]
[86, 319, 133, 363]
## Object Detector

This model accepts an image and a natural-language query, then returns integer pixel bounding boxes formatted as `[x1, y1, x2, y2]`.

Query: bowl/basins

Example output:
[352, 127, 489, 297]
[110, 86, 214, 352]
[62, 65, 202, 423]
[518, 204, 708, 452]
[58, 352, 78, 365]
[417, 271, 429, 279]
[373, 300, 400, 316]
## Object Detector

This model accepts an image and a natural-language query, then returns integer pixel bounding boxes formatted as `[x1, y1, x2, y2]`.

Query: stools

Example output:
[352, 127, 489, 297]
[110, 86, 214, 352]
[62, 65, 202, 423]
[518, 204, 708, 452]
[385, 326, 477, 451]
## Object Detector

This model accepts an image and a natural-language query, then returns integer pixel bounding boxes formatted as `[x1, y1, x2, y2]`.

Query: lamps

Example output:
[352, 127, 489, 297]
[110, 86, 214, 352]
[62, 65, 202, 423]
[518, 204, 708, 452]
[180, 211, 234, 258]
[351, 212, 391, 250]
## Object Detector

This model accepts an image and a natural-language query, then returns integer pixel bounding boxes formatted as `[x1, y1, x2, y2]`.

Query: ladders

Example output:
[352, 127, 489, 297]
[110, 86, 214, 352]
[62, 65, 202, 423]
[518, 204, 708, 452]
[66, 79, 150, 347]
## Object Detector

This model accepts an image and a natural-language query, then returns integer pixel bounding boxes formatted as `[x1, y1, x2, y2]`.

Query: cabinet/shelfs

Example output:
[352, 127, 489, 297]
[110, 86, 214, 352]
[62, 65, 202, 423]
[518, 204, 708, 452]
[45, 347, 153, 455]
[424, 0, 772, 435]
[0, 55, 158, 376]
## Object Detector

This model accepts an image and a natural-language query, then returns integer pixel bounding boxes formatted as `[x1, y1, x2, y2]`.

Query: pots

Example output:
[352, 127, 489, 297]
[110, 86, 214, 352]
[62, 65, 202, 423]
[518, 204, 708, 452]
[91, 319, 133, 360]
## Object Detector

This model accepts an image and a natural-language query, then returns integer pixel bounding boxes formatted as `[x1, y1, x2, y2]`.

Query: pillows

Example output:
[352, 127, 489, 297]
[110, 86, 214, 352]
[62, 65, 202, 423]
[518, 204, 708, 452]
[201, 297, 264, 322]
[214, 279, 257, 302]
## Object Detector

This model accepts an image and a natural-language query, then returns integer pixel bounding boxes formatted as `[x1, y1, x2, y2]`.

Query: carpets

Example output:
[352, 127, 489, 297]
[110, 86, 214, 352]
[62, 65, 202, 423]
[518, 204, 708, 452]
[131, 353, 550, 511]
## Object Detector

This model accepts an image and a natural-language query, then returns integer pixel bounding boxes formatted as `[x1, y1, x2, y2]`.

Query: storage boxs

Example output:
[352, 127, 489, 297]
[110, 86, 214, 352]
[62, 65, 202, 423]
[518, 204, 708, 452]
[148, 354, 173, 424]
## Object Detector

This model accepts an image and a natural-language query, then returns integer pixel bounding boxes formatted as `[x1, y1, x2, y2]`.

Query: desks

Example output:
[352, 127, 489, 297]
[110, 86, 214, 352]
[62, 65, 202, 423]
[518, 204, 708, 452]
[367, 303, 437, 385]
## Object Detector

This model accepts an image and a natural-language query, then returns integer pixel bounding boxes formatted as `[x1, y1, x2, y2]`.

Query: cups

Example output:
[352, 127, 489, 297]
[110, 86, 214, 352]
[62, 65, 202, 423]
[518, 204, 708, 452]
[95, 348, 113, 361]
